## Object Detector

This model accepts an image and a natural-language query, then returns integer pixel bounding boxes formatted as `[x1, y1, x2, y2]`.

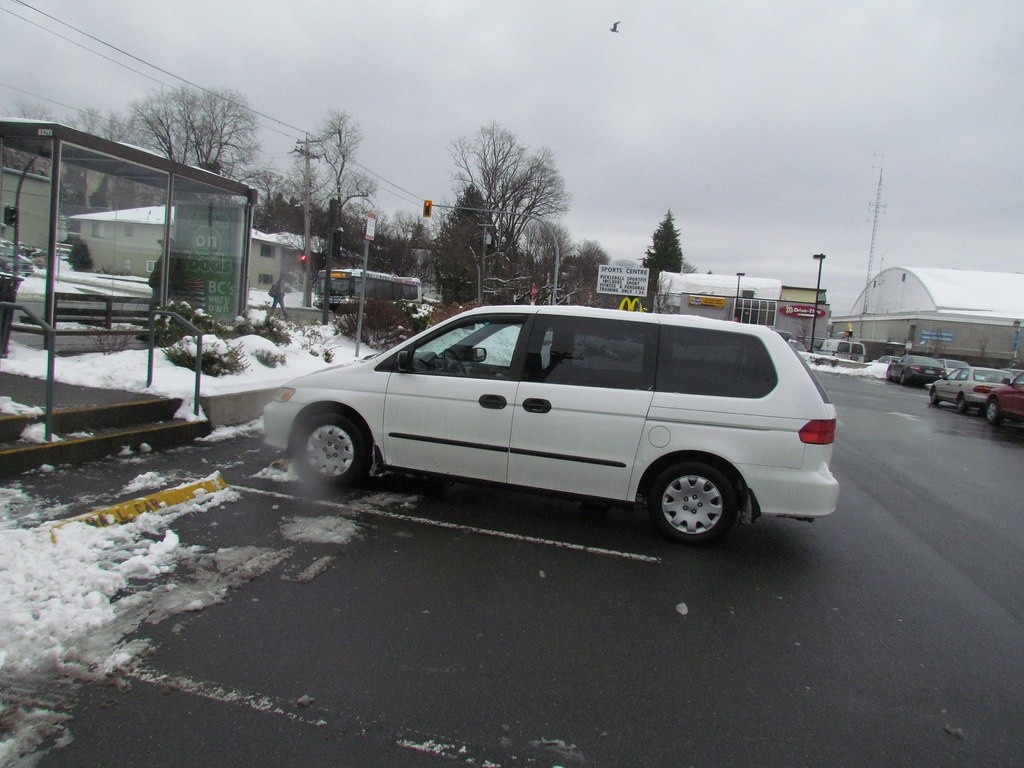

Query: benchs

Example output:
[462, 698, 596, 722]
[20, 293, 152, 329]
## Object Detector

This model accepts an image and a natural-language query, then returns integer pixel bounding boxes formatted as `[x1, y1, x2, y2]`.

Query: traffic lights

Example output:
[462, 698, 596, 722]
[423, 200, 432, 218]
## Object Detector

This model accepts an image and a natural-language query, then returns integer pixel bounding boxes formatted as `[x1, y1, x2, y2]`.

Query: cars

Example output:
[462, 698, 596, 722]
[936, 359, 970, 378]
[983, 371, 1024, 426]
[929, 367, 1024, 417]
[886, 354, 947, 385]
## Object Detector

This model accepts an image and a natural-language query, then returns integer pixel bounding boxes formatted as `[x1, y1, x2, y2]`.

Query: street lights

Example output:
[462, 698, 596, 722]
[807, 253, 826, 353]
[733, 272, 745, 322]
[501, 236, 507, 268]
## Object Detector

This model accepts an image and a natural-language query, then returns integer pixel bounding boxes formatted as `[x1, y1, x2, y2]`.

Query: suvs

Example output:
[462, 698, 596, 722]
[258, 303, 842, 545]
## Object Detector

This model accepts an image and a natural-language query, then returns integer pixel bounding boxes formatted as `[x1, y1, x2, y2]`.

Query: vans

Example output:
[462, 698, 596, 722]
[814, 339, 868, 363]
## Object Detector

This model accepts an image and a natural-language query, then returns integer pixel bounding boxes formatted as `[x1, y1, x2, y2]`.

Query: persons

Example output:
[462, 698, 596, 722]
[136, 238, 176, 340]
[271, 278, 289, 319]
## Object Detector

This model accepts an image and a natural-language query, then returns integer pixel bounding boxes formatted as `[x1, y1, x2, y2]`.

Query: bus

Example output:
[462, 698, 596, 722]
[312, 268, 423, 315]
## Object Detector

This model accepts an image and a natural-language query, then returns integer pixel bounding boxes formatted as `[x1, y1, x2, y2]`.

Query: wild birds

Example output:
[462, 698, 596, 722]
[610, 21, 621, 33]
